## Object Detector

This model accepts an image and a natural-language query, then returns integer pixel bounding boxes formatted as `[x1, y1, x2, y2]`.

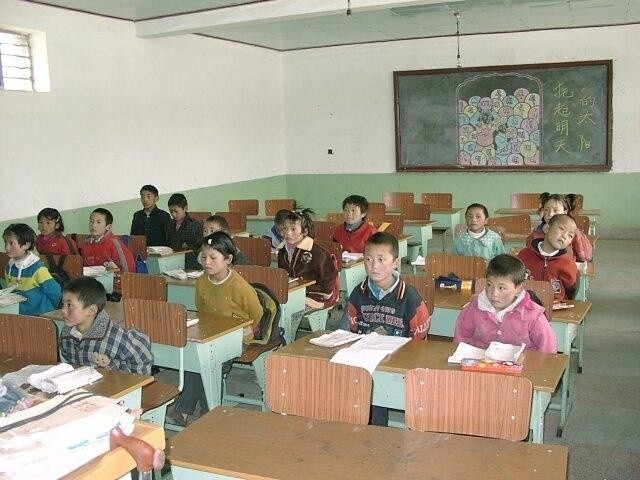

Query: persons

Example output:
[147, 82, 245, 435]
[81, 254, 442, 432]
[78, 208, 138, 287]
[164, 193, 205, 270]
[164, 231, 264, 425]
[36, 208, 81, 256]
[451, 203, 506, 262]
[258, 209, 292, 253]
[129, 184, 172, 249]
[336, 231, 431, 342]
[53, 276, 155, 379]
[200, 215, 252, 263]
[2, 223, 63, 315]
[515, 213, 580, 305]
[453, 252, 559, 354]
[331, 195, 379, 253]
[278, 207, 334, 344]
[526, 192, 592, 262]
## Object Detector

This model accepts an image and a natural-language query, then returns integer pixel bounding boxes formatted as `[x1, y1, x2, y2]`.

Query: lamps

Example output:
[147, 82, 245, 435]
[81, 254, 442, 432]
[344, 0, 353, 24]
[453, 10, 464, 69]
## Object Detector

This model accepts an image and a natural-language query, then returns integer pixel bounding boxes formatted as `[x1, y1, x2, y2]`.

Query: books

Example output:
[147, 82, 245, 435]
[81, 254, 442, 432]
[80, 262, 111, 277]
[1, 287, 29, 308]
[146, 245, 174, 258]
[25, 364, 105, 394]
[447, 340, 526, 364]
[165, 266, 205, 284]
[309, 328, 413, 376]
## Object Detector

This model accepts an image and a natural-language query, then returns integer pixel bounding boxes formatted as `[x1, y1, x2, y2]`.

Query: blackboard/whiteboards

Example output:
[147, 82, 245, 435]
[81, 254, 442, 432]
[393, 58, 612, 174]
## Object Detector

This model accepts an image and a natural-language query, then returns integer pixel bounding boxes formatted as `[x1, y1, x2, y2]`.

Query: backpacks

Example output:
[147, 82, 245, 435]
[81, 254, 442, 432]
[324, 253, 340, 306]
[45, 254, 70, 307]
[133, 256, 149, 275]
[248, 282, 288, 353]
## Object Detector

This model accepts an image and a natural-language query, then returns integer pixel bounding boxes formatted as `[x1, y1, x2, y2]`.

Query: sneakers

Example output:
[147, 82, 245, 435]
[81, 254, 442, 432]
[164, 412, 188, 427]
